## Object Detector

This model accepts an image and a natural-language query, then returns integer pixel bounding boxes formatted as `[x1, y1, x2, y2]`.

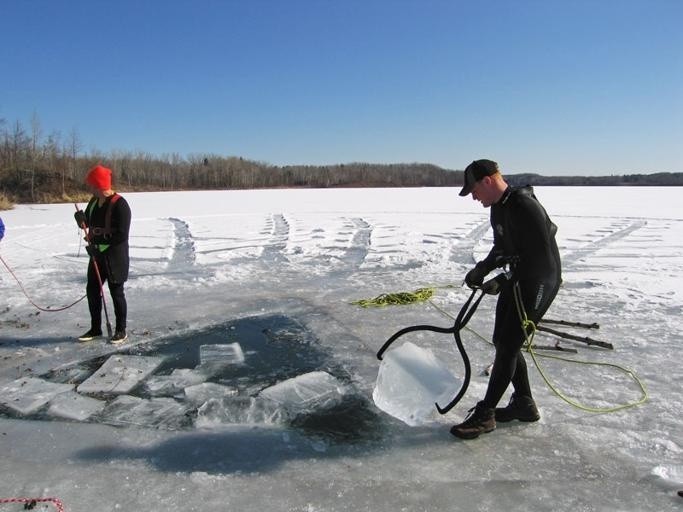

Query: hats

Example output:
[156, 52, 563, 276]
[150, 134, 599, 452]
[86, 166, 112, 189]
[459, 160, 499, 196]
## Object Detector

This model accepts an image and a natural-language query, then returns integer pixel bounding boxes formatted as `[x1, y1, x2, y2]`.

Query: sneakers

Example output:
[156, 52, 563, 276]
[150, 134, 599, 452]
[110, 329, 127, 343]
[77, 330, 102, 341]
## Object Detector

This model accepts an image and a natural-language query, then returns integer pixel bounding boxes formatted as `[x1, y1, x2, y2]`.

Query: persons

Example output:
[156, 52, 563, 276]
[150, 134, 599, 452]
[74, 165, 132, 344]
[450, 158, 563, 440]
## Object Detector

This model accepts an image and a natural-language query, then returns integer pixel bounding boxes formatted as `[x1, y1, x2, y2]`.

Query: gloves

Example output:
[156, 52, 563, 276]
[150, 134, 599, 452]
[75, 210, 88, 228]
[481, 272, 509, 295]
[86, 243, 101, 256]
[465, 266, 483, 288]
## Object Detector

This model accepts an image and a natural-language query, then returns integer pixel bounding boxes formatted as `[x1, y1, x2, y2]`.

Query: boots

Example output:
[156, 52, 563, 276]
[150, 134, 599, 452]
[496, 391, 540, 422]
[450, 401, 497, 439]
